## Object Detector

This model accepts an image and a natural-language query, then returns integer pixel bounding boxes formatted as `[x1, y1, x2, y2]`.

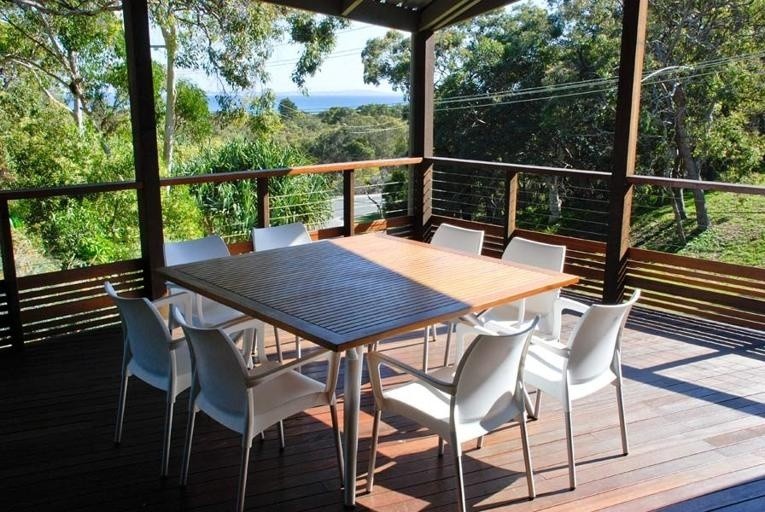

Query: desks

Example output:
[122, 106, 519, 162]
[155, 232, 582, 504]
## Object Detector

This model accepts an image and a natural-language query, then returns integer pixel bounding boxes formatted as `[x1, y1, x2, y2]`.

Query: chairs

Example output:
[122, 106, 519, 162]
[160, 235, 266, 369]
[175, 304, 356, 512]
[376, 224, 483, 373]
[105, 280, 254, 475]
[476, 287, 641, 491]
[367, 315, 543, 512]
[250, 221, 337, 363]
[455, 236, 567, 419]
